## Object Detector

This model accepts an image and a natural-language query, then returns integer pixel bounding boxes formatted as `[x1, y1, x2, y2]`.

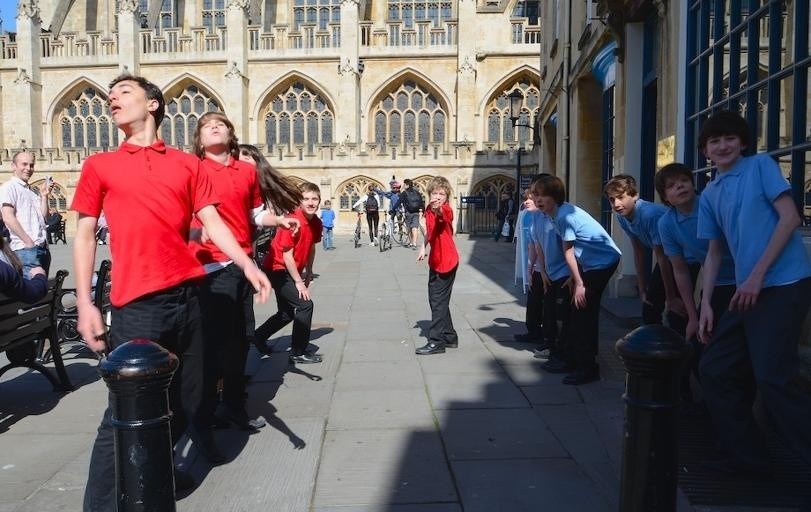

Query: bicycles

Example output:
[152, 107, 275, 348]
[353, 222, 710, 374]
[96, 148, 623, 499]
[379, 209, 397, 251]
[349, 209, 367, 248]
[392, 210, 425, 244]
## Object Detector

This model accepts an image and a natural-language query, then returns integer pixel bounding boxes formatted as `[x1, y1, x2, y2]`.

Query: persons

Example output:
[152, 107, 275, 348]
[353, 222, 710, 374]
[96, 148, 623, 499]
[533, 176, 622, 387]
[375, 181, 405, 242]
[250, 181, 322, 363]
[96, 209, 108, 245]
[0, 216, 49, 302]
[230, 145, 303, 231]
[352, 184, 381, 247]
[73, 73, 269, 512]
[389, 179, 425, 248]
[186, 110, 301, 429]
[416, 175, 461, 356]
[529, 174, 574, 369]
[0, 151, 53, 284]
[493, 192, 513, 242]
[514, 188, 549, 346]
[319, 200, 336, 250]
[655, 163, 737, 350]
[698, 111, 811, 487]
[604, 174, 702, 340]
[47, 209, 63, 245]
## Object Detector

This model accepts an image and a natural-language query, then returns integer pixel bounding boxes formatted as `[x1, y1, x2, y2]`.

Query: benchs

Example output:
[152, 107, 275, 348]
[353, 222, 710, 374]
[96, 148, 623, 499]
[0, 269, 74, 393]
[47, 218, 67, 245]
[43, 259, 112, 363]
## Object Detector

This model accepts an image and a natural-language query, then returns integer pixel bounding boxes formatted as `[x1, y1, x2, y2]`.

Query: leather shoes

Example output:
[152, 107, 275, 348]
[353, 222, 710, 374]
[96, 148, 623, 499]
[532, 348, 550, 359]
[541, 355, 562, 373]
[536, 342, 549, 352]
[288, 352, 322, 365]
[414, 340, 447, 356]
[251, 335, 272, 355]
[446, 336, 459, 348]
[218, 401, 265, 431]
[563, 363, 600, 386]
[514, 331, 542, 342]
